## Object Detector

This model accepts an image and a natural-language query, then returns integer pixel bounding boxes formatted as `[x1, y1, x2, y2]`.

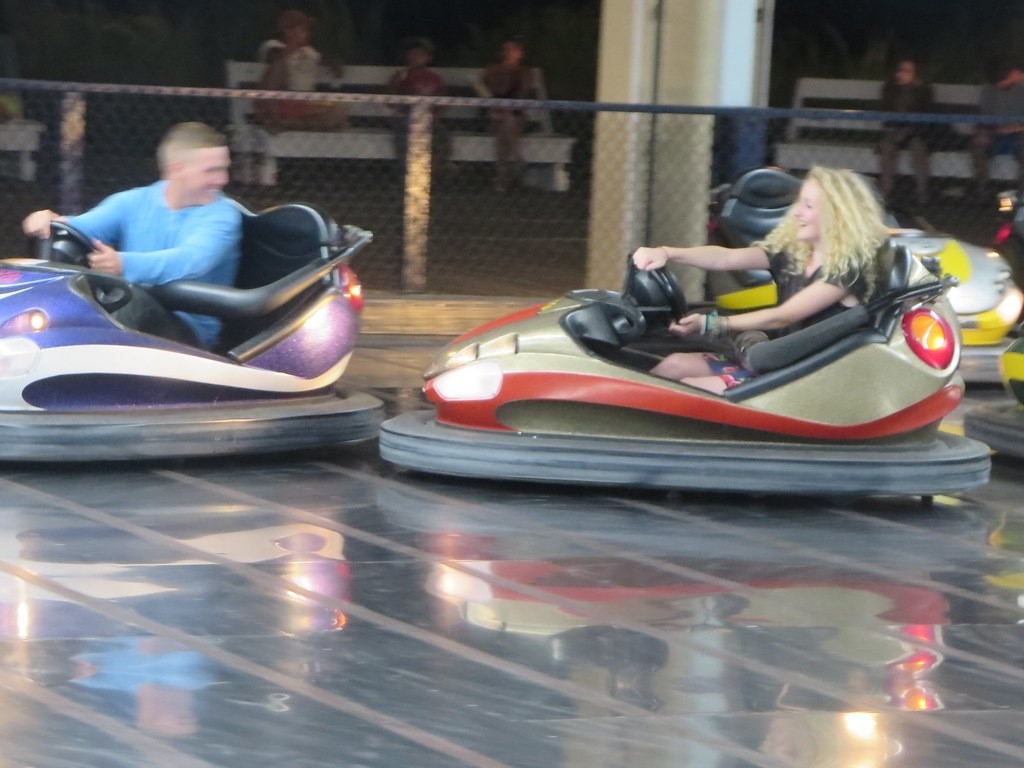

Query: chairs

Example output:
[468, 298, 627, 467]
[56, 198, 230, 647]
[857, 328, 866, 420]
[721, 169, 803, 286]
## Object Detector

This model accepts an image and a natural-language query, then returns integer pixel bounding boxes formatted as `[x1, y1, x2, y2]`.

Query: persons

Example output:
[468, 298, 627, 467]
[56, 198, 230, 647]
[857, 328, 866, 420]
[967, 58, 1024, 207]
[477, 35, 537, 198]
[876, 59, 932, 230]
[630, 166, 891, 395]
[254, 13, 347, 131]
[387, 41, 452, 198]
[22, 123, 243, 352]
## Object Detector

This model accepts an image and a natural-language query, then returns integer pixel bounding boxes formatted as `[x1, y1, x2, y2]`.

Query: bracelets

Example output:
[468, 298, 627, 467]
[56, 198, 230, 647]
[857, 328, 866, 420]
[701, 312, 732, 342]
[659, 245, 672, 262]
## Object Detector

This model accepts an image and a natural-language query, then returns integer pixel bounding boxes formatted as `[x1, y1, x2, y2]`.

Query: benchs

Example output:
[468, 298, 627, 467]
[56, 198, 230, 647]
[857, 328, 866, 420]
[773, 76, 1024, 180]
[226, 59, 577, 192]
